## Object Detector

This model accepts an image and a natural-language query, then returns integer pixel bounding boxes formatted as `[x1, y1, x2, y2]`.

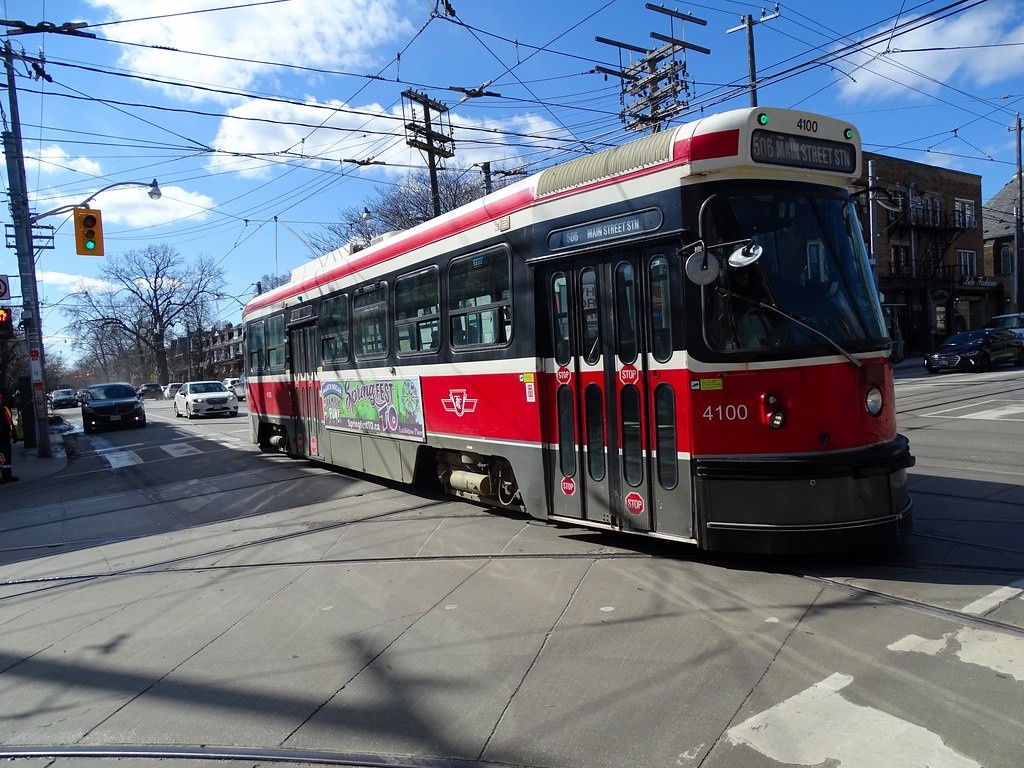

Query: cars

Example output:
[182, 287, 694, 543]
[49, 388, 81, 410]
[172, 381, 238, 418]
[222, 378, 245, 401]
[990, 313, 1024, 343]
[162, 383, 184, 399]
[134, 382, 163, 402]
[159, 384, 169, 393]
[74, 388, 87, 403]
[77, 383, 147, 434]
[923, 329, 1024, 375]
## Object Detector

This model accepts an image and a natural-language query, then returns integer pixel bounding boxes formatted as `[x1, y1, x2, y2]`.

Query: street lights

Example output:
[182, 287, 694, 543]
[362, 207, 406, 230]
[213, 292, 244, 309]
[29, 177, 162, 379]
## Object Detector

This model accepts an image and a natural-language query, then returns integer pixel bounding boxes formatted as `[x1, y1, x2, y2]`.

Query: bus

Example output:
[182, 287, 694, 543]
[241, 105, 919, 566]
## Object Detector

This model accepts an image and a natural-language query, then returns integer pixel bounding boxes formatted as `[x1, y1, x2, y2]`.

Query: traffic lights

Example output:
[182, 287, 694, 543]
[73, 207, 104, 256]
